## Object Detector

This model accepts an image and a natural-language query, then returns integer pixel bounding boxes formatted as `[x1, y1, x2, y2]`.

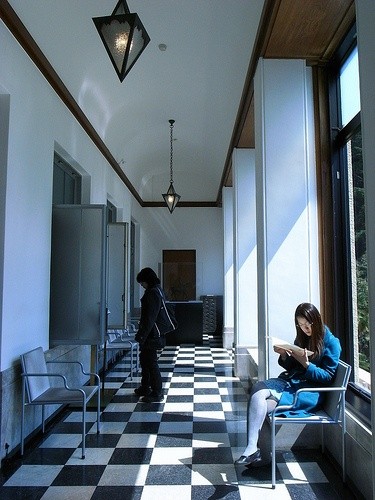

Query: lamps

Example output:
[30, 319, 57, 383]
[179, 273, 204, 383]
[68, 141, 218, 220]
[91, 0, 151, 84]
[160, 118, 181, 216]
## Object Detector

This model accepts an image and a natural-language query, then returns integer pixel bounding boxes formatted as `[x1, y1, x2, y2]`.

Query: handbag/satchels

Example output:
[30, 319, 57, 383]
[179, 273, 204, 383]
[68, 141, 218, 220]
[152, 288, 179, 338]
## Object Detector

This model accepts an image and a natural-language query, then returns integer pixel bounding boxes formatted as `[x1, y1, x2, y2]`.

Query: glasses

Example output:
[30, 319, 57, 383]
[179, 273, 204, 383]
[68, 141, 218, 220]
[297, 321, 314, 328]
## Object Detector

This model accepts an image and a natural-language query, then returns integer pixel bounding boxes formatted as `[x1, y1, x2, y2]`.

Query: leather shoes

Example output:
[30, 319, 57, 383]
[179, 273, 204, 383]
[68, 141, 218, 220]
[234, 448, 262, 465]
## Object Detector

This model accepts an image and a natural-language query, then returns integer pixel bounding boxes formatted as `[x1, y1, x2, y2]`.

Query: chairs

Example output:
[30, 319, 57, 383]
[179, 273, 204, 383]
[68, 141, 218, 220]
[268, 360, 351, 489]
[103, 317, 140, 380]
[19, 347, 100, 460]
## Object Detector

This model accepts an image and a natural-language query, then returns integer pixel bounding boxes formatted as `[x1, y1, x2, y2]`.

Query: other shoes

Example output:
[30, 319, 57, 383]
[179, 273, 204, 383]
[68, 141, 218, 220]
[134, 386, 154, 394]
[142, 391, 164, 403]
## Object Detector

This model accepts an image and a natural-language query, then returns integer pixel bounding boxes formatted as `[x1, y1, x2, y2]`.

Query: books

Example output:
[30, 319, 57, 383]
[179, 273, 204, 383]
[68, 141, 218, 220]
[266, 335, 315, 358]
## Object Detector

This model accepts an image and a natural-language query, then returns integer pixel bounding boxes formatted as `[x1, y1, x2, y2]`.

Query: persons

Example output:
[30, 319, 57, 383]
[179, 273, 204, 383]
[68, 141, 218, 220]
[133, 268, 175, 403]
[233, 302, 342, 467]
